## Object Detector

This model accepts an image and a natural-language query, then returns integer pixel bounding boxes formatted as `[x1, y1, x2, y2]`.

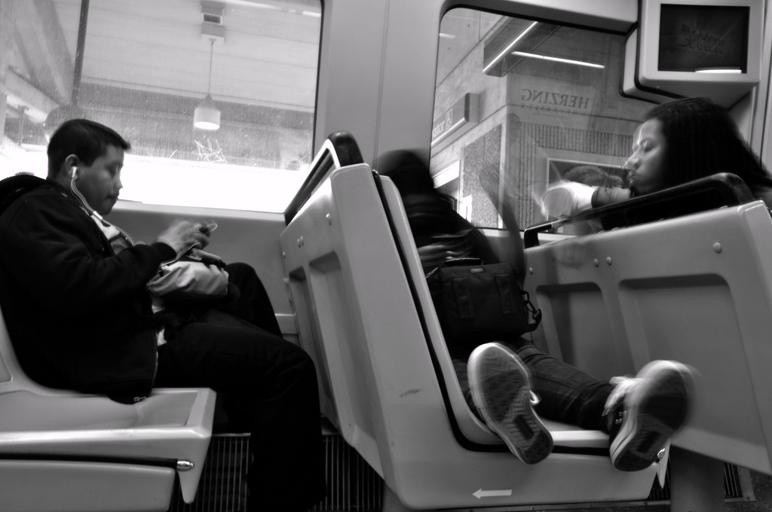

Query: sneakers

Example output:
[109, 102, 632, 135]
[606, 359, 693, 472]
[466, 340, 553, 466]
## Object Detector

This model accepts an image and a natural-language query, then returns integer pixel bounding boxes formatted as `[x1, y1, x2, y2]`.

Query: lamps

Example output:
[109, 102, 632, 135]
[191, 22, 227, 130]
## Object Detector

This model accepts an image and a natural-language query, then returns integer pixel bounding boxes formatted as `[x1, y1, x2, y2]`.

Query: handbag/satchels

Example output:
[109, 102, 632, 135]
[424, 261, 542, 351]
[147, 242, 231, 299]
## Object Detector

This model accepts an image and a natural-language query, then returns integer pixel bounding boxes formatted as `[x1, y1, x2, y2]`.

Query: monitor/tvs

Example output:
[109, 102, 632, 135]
[639, 0, 762, 103]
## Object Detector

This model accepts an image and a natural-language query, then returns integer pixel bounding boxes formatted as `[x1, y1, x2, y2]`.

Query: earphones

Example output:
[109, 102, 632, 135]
[71, 166, 78, 181]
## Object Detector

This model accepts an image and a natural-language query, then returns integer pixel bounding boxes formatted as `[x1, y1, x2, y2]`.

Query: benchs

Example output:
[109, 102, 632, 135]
[521, 172, 770, 511]
[279, 130, 672, 511]
[1, 131, 216, 510]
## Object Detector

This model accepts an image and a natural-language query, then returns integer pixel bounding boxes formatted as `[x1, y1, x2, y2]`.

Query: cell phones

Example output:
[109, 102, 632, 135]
[195, 222, 210, 239]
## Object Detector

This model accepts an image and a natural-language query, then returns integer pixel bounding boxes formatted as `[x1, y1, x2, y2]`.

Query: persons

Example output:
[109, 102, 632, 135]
[533, 96, 772, 232]
[0, 118, 330, 511]
[372, 146, 692, 472]
